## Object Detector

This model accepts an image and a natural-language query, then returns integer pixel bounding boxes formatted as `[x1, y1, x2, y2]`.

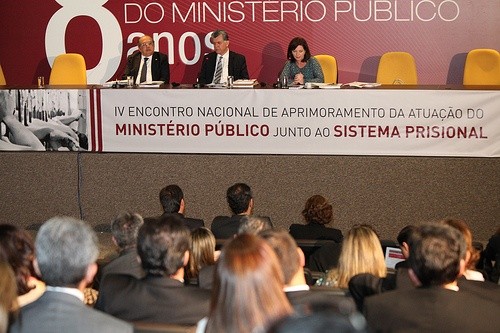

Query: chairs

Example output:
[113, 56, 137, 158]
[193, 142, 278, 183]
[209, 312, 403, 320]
[463, 49, 500, 85]
[297, 239, 335, 264]
[376, 52, 417, 84]
[50, 53, 86, 86]
[313, 54, 337, 85]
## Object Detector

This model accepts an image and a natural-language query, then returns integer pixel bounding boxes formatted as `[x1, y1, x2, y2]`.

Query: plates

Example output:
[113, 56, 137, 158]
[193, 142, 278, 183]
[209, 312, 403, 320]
[303, 87, 321, 89]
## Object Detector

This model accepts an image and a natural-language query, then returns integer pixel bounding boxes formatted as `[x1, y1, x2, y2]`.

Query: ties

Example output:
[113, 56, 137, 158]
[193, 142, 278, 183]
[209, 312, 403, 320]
[214, 57, 223, 84]
[140, 57, 149, 83]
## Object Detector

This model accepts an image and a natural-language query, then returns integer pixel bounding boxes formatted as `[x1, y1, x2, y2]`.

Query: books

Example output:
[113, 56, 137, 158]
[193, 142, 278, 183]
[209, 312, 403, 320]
[140, 81, 164, 87]
[319, 82, 344, 89]
[103, 80, 127, 87]
[232, 79, 258, 88]
[346, 81, 381, 88]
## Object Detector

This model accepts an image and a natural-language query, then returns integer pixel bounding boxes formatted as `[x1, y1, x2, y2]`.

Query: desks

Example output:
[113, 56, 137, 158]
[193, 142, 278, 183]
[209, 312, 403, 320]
[0, 84, 500, 159]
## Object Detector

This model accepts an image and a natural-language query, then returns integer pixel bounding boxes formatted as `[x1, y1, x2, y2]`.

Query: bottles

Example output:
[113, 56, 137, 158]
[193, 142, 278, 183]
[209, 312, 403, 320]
[282, 75, 288, 88]
[275, 78, 281, 88]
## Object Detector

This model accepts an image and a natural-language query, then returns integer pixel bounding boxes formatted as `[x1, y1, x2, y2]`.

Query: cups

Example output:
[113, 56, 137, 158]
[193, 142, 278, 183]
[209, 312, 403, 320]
[305, 82, 311, 88]
[38, 76, 44, 88]
[126, 75, 134, 88]
[227, 75, 234, 88]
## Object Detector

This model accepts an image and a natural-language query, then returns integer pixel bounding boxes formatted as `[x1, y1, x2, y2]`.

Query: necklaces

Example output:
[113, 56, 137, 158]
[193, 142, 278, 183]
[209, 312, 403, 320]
[316, 226, 386, 289]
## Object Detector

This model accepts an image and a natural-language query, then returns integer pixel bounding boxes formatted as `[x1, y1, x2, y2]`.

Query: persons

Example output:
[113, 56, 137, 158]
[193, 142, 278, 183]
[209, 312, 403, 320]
[279, 37, 325, 84]
[209, 232, 371, 333]
[364, 223, 500, 333]
[436, 218, 485, 281]
[288, 194, 343, 240]
[256, 227, 344, 296]
[0, 223, 45, 333]
[485, 228, 500, 283]
[10, 215, 134, 332]
[159, 182, 274, 287]
[0, 91, 85, 153]
[123, 35, 170, 84]
[200, 30, 249, 84]
[96, 213, 217, 326]
